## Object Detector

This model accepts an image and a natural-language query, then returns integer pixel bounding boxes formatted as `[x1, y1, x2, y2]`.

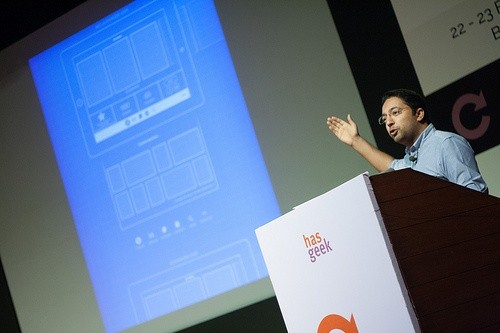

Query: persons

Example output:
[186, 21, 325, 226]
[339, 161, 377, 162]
[327, 89, 490, 195]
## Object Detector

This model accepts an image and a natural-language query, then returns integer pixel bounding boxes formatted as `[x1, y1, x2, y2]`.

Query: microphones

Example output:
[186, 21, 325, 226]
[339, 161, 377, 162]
[408, 156, 417, 162]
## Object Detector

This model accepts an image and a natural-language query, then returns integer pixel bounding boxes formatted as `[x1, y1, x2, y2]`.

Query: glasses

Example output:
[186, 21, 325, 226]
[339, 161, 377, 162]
[381, 107, 409, 121]
